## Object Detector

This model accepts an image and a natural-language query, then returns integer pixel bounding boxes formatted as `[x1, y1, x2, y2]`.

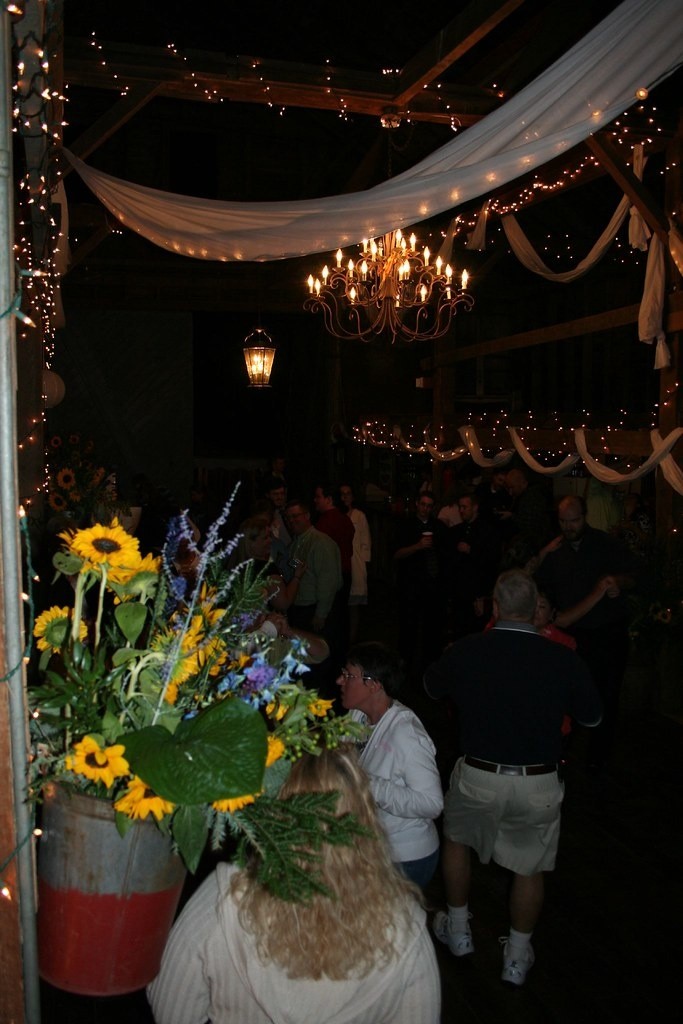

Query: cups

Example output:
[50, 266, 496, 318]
[423, 531, 433, 546]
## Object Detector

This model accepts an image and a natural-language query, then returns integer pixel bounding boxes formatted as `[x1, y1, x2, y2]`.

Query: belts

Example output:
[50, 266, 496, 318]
[464, 754, 560, 776]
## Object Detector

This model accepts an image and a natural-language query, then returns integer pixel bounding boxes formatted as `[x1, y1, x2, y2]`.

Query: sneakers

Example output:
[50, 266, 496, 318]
[498, 935, 535, 984]
[433, 908, 476, 958]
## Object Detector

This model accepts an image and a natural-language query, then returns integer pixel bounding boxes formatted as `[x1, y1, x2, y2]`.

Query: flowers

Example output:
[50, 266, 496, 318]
[14, 478, 377, 910]
[42, 430, 127, 524]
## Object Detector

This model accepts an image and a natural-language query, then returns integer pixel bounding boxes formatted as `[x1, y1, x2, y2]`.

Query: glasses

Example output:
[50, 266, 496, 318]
[340, 667, 372, 684]
[283, 511, 308, 521]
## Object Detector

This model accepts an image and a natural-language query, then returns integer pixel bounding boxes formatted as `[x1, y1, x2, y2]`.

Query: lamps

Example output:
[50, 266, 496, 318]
[241, 323, 276, 389]
[303, 208, 474, 342]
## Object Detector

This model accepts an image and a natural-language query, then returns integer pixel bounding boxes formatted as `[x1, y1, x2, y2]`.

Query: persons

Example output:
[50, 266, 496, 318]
[23, 415, 654, 736]
[147, 745, 442, 1024]
[331, 645, 444, 894]
[424, 569, 605, 985]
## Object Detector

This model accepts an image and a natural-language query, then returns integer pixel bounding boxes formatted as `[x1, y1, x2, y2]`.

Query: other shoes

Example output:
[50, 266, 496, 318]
[585, 737, 610, 777]
